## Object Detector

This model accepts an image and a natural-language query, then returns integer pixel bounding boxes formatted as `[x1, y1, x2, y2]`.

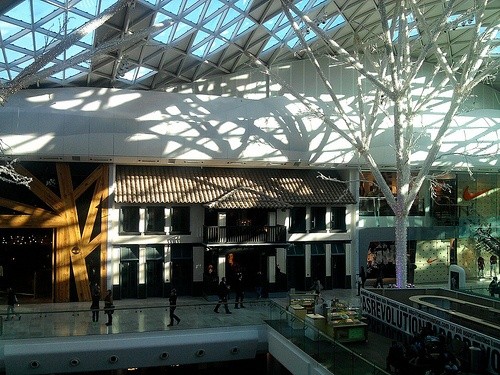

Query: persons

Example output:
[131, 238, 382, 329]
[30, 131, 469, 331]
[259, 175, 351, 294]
[3, 288, 21, 321]
[490, 254, 498, 279]
[372, 265, 384, 288]
[167, 289, 180, 327]
[386, 325, 464, 375]
[360, 265, 367, 291]
[104, 290, 114, 325]
[356, 273, 362, 296]
[235, 274, 245, 308]
[91, 286, 101, 322]
[489, 277, 500, 299]
[214, 277, 232, 314]
[478, 256, 485, 277]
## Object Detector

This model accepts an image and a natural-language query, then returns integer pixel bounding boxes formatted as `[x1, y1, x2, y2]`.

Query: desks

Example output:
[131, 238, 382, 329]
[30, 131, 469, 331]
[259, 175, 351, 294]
[408, 295, 500, 330]
[288, 293, 318, 313]
[325, 306, 360, 312]
[304, 314, 327, 341]
[288, 305, 307, 329]
[327, 319, 369, 344]
[330, 315, 367, 322]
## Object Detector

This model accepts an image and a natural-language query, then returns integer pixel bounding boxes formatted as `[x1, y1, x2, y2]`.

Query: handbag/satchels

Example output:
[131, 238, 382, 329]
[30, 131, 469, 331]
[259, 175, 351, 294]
[358, 276, 362, 284]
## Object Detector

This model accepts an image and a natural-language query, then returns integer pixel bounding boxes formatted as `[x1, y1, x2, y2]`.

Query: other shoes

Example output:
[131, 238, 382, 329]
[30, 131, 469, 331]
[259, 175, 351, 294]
[177, 319, 180, 325]
[357, 295, 360, 296]
[168, 324, 173, 326]
[106, 323, 112, 326]
[5, 319, 9, 321]
[19, 316, 21, 321]
[235, 307, 240, 309]
[226, 312, 232, 314]
[214, 310, 219, 313]
[240, 306, 245, 308]
